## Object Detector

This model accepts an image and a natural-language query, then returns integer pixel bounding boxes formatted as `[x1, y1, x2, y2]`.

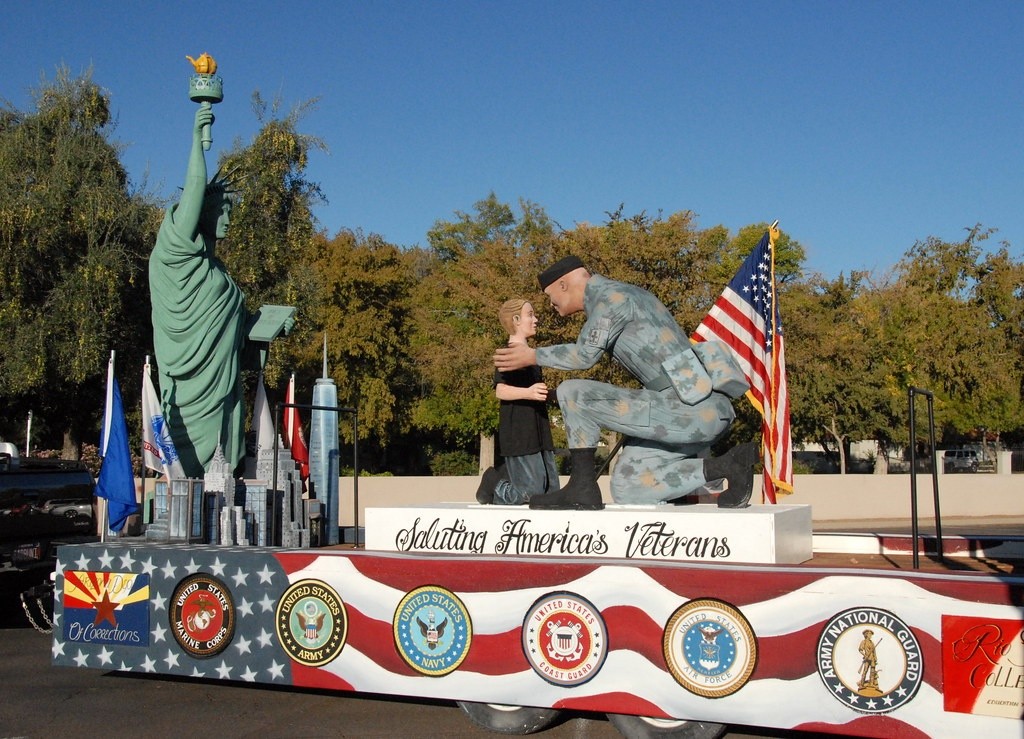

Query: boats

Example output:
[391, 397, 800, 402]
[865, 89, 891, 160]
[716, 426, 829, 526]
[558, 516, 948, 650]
[48, 51, 1024, 738]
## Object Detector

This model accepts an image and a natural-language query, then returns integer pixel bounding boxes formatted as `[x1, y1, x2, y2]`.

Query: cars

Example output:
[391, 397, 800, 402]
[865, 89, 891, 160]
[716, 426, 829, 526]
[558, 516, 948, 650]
[945, 450, 980, 473]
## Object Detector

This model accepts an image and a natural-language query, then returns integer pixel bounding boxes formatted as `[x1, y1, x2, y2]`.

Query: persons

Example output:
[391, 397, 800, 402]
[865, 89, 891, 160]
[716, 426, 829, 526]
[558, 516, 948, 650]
[476, 298, 559, 504]
[493, 255, 759, 510]
[149, 105, 247, 480]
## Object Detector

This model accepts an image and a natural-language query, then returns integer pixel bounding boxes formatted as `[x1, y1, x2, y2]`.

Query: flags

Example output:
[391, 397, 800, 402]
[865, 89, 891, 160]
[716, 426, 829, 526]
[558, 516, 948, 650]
[94, 378, 137, 533]
[142, 368, 185, 486]
[689, 230, 794, 505]
[284, 383, 310, 494]
